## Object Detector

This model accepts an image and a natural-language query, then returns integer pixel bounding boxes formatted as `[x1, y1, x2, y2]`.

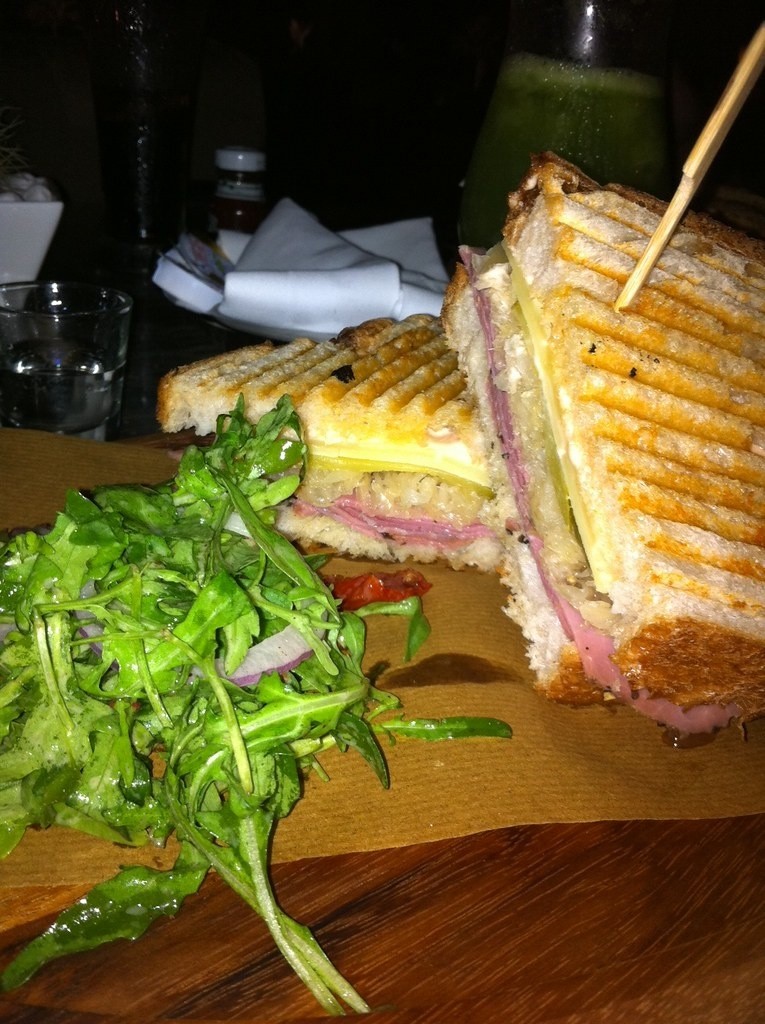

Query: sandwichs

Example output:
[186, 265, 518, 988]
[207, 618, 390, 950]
[161, 313, 505, 574]
[439, 155, 765, 735]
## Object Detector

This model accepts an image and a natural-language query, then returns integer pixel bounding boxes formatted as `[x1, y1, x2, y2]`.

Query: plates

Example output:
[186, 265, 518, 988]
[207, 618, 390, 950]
[167, 244, 337, 343]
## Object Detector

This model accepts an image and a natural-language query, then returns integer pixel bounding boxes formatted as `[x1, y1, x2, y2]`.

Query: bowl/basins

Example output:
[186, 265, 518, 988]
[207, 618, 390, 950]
[0, 199, 67, 312]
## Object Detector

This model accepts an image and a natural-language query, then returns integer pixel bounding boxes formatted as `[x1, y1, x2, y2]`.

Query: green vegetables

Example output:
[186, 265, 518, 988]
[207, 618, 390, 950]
[0, 390, 514, 1014]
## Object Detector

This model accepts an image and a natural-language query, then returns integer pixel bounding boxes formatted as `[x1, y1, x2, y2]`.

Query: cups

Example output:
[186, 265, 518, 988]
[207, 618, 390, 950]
[83, 0, 203, 245]
[0, 282, 134, 446]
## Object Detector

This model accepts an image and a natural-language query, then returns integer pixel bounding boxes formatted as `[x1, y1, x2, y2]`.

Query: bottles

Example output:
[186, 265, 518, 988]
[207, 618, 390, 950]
[457, 0, 679, 268]
[188, 143, 271, 345]
